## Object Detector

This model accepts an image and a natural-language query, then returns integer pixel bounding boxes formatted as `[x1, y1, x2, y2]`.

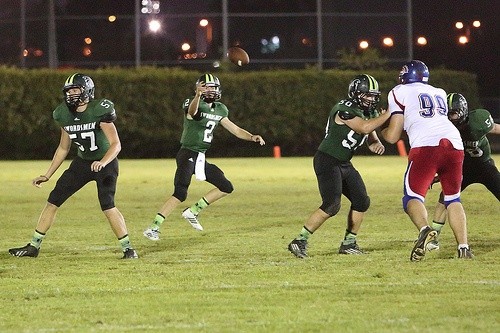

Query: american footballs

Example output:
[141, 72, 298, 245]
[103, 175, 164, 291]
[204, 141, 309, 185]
[226, 46, 251, 67]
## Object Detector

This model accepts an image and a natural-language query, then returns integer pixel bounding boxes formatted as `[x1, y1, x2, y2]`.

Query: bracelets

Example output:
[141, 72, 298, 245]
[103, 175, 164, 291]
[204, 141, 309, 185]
[42, 176, 50, 180]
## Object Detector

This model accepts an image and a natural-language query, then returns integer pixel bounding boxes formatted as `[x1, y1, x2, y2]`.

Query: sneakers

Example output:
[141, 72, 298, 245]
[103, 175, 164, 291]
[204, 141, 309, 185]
[338, 240, 364, 254]
[8, 243, 40, 257]
[409, 225, 437, 262]
[286, 237, 308, 259]
[121, 247, 139, 259]
[457, 244, 476, 258]
[181, 206, 204, 231]
[143, 228, 162, 241]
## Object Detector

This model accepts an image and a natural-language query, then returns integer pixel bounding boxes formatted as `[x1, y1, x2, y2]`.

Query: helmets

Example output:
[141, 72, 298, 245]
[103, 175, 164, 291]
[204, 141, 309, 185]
[63, 73, 96, 111]
[447, 93, 470, 126]
[192, 73, 222, 104]
[348, 74, 382, 117]
[398, 59, 430, 84]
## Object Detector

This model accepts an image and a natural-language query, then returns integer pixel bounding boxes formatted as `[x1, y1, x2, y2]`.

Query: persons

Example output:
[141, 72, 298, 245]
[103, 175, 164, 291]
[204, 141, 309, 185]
[143, 73, 265, 240]
[288, 74, 390, 259]
[9, 73, 138, 258]
[380, 61, 473, 263]
[430, 93, 500, 235]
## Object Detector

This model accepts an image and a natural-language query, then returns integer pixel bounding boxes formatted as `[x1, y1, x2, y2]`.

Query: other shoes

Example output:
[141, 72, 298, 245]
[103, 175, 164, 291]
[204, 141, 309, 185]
[427, 240, 439, 252]
